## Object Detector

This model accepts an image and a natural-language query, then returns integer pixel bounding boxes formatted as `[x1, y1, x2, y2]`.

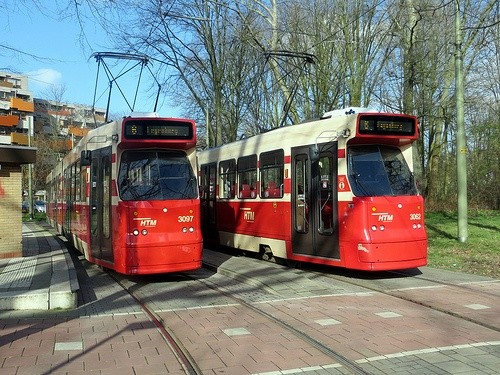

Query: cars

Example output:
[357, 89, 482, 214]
[22, 201, 28, 213]
[33, 200, 46, 214]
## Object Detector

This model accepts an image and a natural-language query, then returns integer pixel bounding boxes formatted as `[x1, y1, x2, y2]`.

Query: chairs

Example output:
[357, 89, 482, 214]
[230, 181, 332, 215]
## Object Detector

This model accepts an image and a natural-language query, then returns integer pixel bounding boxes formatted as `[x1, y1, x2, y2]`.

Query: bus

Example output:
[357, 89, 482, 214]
[193, 48, 428, 270]
[46, 51, 205, 275]
